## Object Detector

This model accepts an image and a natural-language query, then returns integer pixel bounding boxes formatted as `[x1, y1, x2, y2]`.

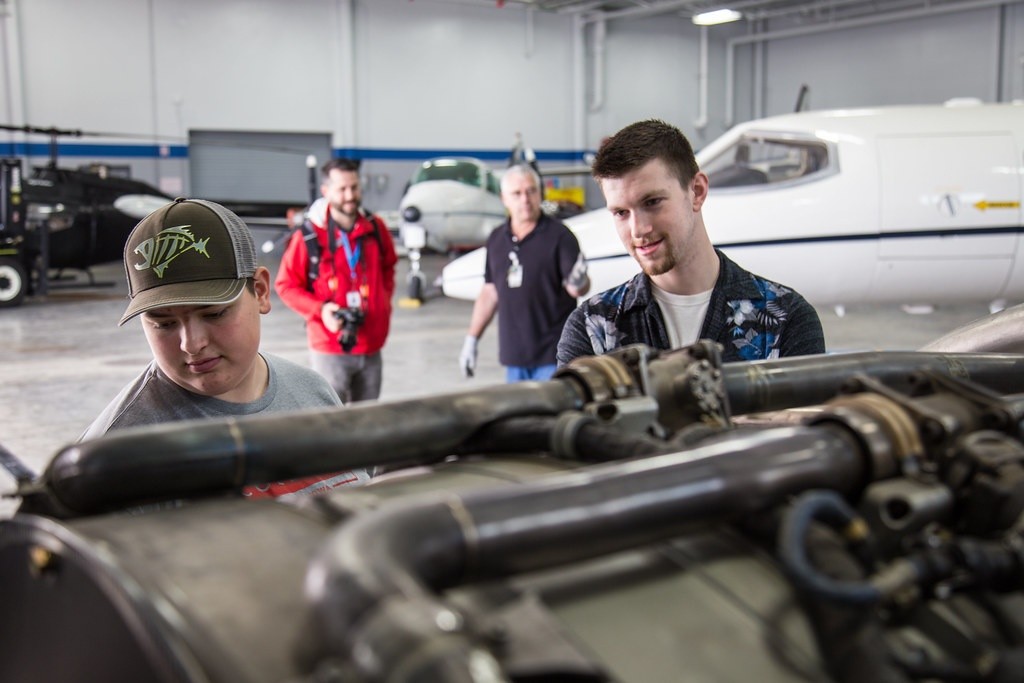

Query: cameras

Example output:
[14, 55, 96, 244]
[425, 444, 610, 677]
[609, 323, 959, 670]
[332, 306, 366, 353]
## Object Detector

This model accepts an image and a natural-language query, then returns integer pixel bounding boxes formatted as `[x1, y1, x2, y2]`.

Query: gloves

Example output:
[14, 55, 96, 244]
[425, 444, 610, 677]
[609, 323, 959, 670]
[565, 252, 589, 291]
[460, 335, 478, 379]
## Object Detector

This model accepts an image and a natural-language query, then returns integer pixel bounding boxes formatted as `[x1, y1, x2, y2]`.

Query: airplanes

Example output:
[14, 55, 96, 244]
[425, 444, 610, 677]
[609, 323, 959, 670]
[441, 96, 1024, 354]
[240, 154, 557, 258]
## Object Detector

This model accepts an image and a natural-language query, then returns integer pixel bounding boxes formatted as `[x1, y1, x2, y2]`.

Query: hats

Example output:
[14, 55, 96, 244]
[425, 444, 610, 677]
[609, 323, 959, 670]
[117, 197, 259, 327]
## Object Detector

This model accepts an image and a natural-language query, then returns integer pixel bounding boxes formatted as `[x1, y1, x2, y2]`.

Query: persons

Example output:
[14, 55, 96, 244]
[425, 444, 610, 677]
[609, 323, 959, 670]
[77, 198, 343, 443]
[555, 119, 825, 369]
[276, 160, 399, 404]
[458, 164, 590, 381]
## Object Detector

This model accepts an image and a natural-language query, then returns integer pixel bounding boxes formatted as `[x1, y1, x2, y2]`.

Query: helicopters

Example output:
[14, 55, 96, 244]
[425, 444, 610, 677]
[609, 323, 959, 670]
[0, 124, 315, 308]
